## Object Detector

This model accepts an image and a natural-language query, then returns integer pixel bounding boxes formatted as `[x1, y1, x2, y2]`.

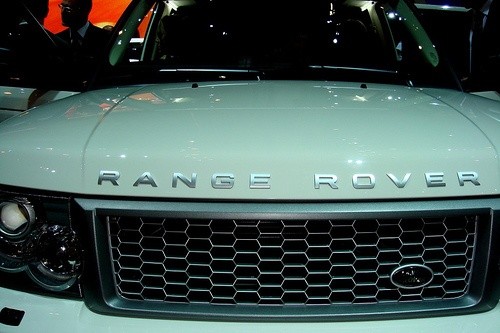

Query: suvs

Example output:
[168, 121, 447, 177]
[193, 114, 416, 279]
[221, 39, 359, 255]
[1, 0, 499, 331]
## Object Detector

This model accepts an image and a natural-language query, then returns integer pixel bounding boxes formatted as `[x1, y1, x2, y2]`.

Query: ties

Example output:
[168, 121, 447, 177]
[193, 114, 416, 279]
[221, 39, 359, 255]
[72, 32, 81, 54]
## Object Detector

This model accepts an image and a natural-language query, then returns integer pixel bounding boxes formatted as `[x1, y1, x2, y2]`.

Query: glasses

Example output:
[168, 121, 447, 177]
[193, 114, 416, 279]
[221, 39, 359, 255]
[63, 6, 82, 13]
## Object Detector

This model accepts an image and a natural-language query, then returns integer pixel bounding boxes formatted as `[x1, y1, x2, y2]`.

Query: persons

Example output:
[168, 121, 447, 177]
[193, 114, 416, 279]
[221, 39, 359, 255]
[0, 0, 116, 89]
